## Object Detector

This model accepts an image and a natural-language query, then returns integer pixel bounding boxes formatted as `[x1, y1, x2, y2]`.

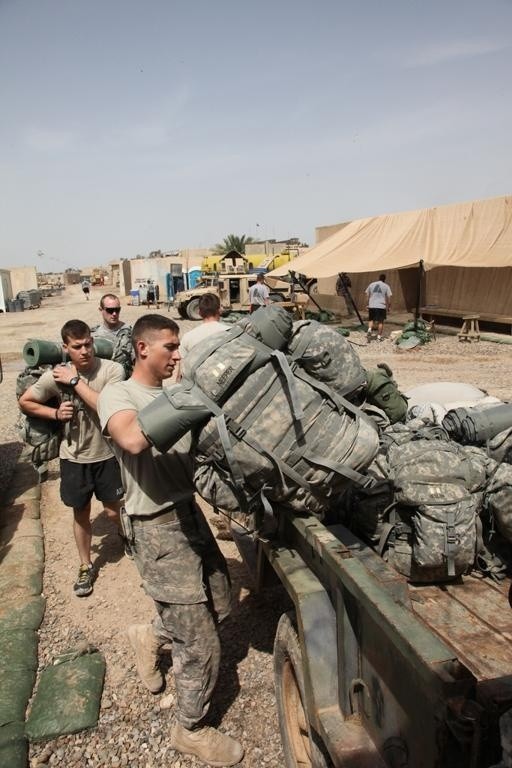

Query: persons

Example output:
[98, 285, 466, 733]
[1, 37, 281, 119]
[88, 293, 133, 346]
[96, 316, 249, 767]
[82, 278, 90, 301]
[364, 273, 392, 342]
[145, 279, 156, 309]
[335, 272, 354, 315]
[249, 273, 270, 315]
[18, 318, 134, 597]
[175, 292, 231, 385]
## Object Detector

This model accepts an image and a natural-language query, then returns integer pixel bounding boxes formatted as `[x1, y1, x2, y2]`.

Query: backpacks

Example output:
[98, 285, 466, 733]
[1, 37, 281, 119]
[137, 303, 382, 514]
[16, 337, 113, 465]
[352, 377, 512, 586]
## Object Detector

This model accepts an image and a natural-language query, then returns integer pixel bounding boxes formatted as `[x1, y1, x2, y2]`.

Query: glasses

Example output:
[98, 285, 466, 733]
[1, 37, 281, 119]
[102, 307, 122, 314]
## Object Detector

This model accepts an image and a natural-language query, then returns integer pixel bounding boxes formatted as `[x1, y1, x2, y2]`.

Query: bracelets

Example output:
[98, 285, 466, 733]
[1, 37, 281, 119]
[55, 409, 59, 421]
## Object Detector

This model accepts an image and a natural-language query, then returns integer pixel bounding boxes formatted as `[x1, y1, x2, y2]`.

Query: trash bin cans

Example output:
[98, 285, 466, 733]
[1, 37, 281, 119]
[131, 289, 140, 305]
[6, 289, 40, 311]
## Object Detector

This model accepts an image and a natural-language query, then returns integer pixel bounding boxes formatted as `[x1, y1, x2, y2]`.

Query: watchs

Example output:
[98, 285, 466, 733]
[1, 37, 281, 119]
[70, 376, 80, 388]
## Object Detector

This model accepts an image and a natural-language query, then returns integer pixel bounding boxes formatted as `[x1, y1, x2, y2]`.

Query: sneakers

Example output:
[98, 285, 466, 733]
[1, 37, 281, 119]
[73, 561, 95, 597]
[168, 722, 246, 766]
[117, 530, 135, 559]
[126, 623, 167, 693]
[366, 331, 383, 342]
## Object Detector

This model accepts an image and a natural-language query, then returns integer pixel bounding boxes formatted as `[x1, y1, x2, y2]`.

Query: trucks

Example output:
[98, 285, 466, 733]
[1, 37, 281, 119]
[173, 272, 317, 321]
